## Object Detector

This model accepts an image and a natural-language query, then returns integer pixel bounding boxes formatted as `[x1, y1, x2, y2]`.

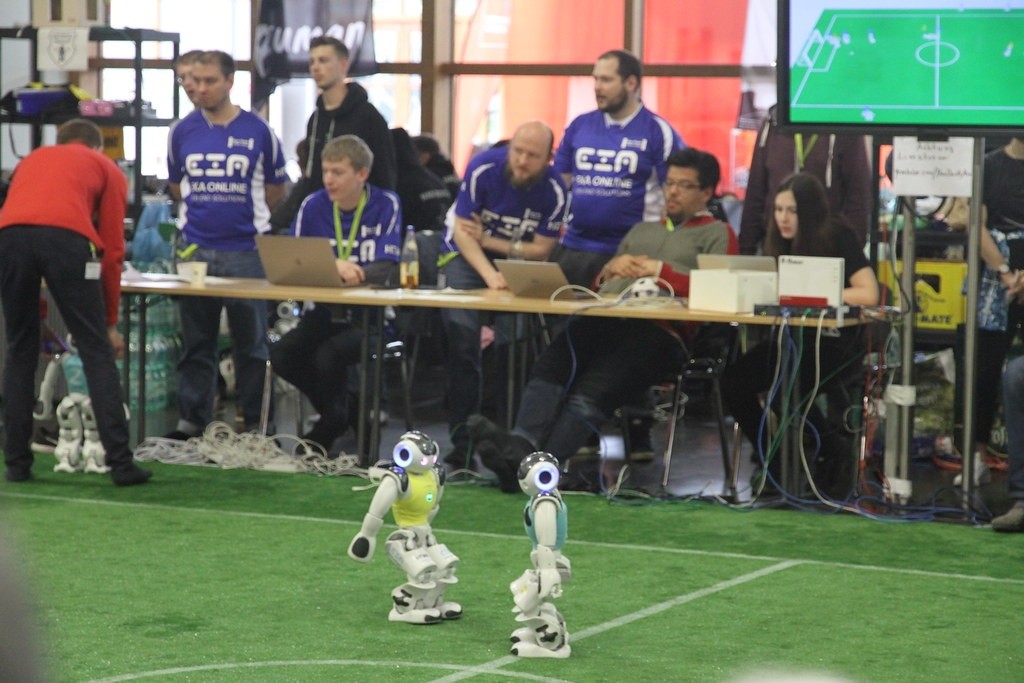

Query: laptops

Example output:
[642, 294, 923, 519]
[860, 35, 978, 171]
[494, 259, 596, 300]
[254, 235, 366, 288]
[697, 253, 775, 273]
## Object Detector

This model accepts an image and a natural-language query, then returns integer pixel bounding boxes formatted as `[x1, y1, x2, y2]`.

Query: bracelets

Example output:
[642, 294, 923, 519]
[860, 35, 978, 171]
[512, 240, 522, 250]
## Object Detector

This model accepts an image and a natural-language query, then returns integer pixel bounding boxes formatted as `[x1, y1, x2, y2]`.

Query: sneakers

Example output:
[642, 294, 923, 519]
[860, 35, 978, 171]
[952, 452, 991, 487]
[753, 475, 780, 493]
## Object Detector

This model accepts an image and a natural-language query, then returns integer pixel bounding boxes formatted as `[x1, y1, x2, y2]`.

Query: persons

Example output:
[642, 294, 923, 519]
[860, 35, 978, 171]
[347, 431, 463, 623]
[934, 130, 1023, 534]
[0, 119, 153, 487]
[509, 451, 571, 658]
[163, 36, 880, 510]
[32, 332, 130, 474]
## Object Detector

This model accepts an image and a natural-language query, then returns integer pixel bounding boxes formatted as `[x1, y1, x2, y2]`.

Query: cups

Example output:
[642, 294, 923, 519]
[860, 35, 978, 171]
[176, 261, 207, 288]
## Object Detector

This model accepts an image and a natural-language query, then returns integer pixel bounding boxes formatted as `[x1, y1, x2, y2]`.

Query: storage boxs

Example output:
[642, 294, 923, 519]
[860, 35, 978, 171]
[687, 255, 849, 314]
[878, 260, 967, 329]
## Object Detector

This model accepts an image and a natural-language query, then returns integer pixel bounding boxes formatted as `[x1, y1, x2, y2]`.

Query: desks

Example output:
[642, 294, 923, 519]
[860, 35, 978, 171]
[42, 273, 893, 504]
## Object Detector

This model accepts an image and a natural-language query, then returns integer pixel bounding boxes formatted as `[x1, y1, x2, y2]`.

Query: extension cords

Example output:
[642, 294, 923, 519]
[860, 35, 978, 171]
[754, 305, 863, 319]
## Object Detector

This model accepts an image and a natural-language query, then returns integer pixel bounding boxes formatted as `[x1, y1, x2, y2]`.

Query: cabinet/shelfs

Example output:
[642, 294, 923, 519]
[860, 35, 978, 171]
[0, 24, 182, 447]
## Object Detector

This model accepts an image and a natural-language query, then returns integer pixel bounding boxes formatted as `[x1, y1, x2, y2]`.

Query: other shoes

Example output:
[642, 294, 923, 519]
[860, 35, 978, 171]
[364, 425, 382, 455]
[6, 470, 37, 482]
[992, 508, 1024, 531]
[466, 413, 526, 493]
[163, 431, 190, 441]
[113, 465, 153, 486]
[442, 449, 478, 467]
[816, 438, 847, 493]
[296, 418, 349, 461]
[267, 434, 281, 448]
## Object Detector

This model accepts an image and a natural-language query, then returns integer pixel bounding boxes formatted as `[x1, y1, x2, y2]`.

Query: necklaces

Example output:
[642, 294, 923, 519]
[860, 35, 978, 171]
[664, 215, 674, 231]
[333, 192, 367, 261]
[794, 133, 818, 168]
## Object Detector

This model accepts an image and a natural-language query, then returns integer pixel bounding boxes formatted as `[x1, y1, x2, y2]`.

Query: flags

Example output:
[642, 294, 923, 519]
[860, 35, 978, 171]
[253, 0, 378, 104]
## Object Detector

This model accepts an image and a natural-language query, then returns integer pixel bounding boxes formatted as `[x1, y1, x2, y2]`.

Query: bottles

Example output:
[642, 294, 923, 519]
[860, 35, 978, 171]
[398, 225, 419, 288]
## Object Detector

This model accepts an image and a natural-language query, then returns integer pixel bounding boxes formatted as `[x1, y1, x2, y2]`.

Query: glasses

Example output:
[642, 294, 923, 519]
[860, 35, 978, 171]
[662, 180, 701, 192]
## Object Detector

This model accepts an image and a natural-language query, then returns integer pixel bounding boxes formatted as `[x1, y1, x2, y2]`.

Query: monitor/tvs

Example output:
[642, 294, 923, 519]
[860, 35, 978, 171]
[777, 1, 1024, 139]
